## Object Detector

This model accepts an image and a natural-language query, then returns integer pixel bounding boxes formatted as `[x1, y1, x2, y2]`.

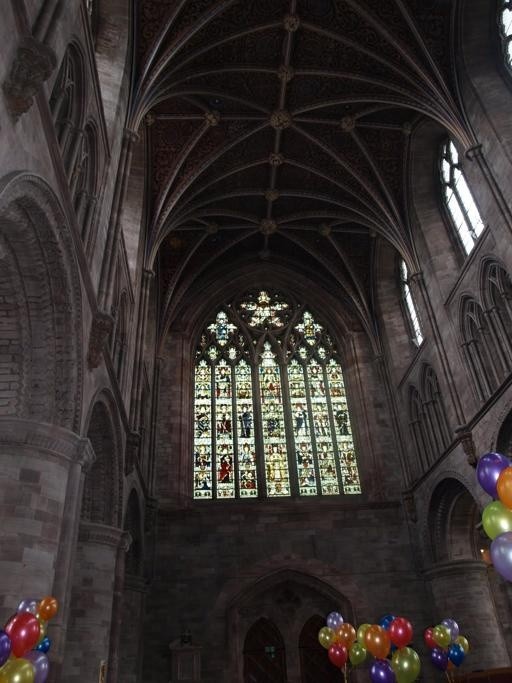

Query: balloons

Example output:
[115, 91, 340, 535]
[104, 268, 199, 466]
[318, 610, 469, 682]
[0, 596, 58, 682]
[475, 452, 512, 585]
